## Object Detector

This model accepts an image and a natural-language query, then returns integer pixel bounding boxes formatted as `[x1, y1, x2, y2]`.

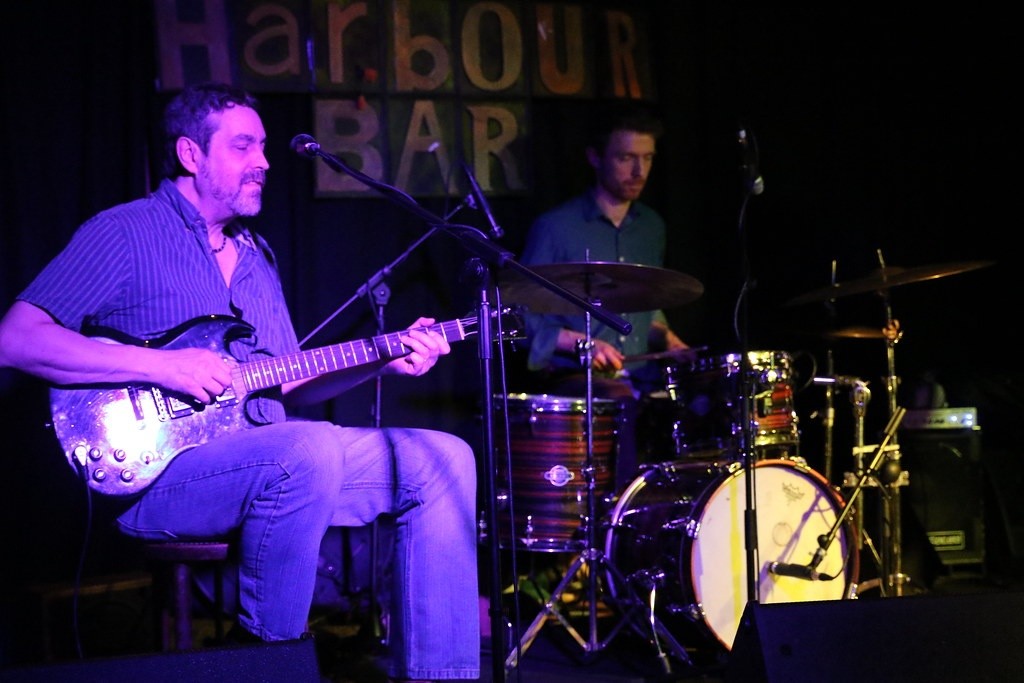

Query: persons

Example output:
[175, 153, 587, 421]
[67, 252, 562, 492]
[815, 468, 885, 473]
[1, 81, 483, 682]
[513, 98, 694, 401]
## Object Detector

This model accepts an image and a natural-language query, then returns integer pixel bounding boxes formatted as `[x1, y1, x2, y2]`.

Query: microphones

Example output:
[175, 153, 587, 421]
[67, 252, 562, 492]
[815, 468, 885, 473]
[769, 562, 833, 582]
[291, 134, 320, 158]
[460, 163, 504, 238]
[738, 129, 763, 194]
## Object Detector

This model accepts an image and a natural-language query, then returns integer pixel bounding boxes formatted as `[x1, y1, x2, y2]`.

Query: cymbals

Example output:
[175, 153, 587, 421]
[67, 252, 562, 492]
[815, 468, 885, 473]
[821, 324, 888, 340]
[479, 257, 709, 319]
[780, 249, 997, 313]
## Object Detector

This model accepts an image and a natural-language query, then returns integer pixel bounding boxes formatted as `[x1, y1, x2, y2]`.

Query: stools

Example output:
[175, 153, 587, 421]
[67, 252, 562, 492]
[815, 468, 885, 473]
[144, 538, 229, 653]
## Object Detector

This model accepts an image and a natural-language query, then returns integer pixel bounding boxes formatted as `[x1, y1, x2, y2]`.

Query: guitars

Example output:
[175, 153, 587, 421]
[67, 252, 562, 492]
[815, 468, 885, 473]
[45, 304, 508, 497]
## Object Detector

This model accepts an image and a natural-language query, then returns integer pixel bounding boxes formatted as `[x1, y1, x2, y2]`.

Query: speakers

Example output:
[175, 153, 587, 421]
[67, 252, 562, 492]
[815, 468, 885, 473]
[0, 636, 321, 683]
[898, 428, 984, 565]
[723, 590, 1024, 683]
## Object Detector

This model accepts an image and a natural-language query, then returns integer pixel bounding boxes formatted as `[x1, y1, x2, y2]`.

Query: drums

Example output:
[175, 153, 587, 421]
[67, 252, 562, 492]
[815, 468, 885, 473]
[484, 391, 627, 556]
[643, 349, 800, 467]
[603, 450, 861, 669]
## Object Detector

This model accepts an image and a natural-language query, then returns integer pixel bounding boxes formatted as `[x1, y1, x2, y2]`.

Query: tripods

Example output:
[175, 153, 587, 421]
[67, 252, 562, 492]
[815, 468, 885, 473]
[499, 297, 693, 680]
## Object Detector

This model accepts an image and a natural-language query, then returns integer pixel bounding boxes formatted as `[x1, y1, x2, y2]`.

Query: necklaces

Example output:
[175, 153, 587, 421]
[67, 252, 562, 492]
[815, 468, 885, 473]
[211, 236, 226, 254]
